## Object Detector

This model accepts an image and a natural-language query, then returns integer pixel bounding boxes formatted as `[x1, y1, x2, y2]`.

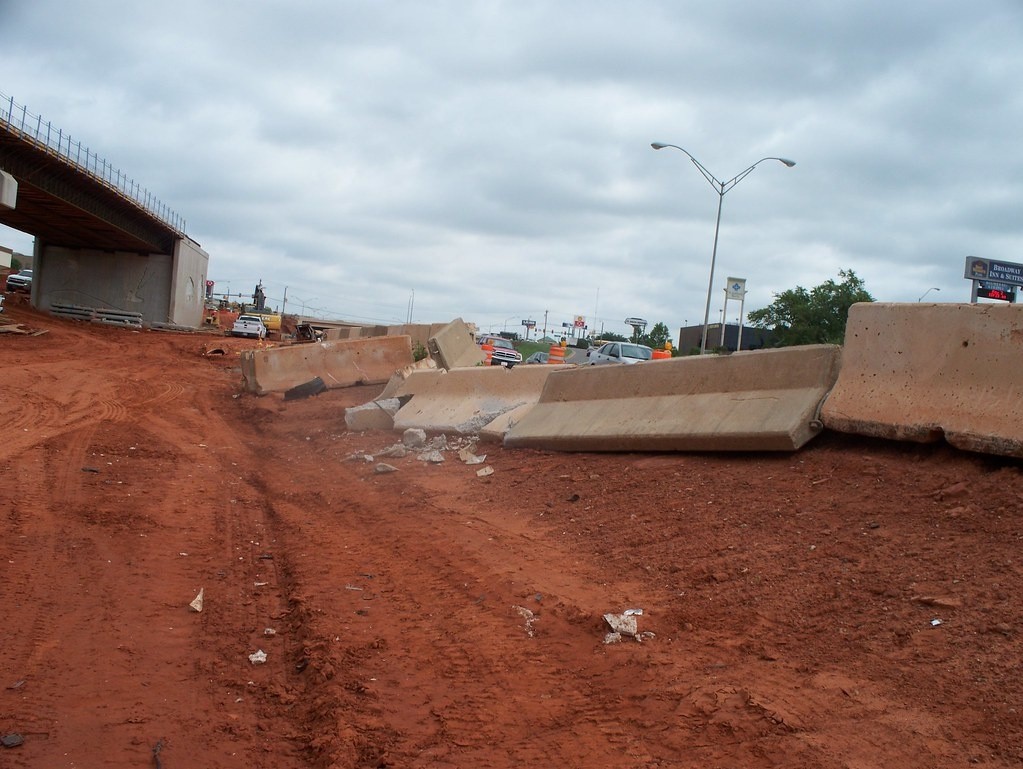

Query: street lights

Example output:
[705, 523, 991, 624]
[407, 289, 414, 324]
[650, 142, 796, 354]
[489, 323, 503, 336]
[504, 316, 520, 334]
[291, 295, 304, 318]
[918, 288, 941, 302]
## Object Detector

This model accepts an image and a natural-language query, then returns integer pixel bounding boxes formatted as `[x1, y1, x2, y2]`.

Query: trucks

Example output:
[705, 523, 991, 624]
[565, 337, 589, 349]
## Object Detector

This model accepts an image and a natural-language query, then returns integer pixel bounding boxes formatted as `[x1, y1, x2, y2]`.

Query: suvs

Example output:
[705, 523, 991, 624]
[477, 335, 524, 369]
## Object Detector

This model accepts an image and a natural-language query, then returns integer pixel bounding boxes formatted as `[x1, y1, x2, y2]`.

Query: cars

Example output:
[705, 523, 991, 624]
[586, 347, 598, 356]
[588, 342, 655, 364]
[6, 269, 33, 293]
[205, 304, 218, 311]
[526, 352, 566, 364]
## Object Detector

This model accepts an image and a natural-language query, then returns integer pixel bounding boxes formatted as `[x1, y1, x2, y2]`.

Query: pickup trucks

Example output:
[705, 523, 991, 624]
[232, 315, 266, 339]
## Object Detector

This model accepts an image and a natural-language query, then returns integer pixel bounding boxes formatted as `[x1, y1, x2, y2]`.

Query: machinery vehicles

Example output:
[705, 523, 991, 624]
[238, 284, 283, 341]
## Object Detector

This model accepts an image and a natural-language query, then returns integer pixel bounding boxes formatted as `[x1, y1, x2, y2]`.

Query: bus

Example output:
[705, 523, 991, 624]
[593, 339, 611, 347]
